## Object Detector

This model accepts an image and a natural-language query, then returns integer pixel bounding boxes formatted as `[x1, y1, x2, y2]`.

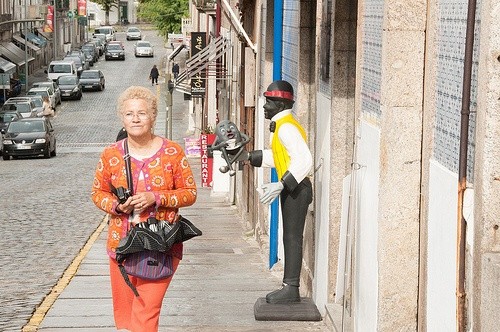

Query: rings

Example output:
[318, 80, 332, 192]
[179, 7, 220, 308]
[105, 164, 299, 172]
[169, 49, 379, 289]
[140, 203, 142, 207]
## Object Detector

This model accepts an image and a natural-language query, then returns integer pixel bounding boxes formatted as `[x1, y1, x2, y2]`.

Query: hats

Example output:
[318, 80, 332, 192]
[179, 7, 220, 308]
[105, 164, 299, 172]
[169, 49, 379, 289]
[263, 80, 295, 102]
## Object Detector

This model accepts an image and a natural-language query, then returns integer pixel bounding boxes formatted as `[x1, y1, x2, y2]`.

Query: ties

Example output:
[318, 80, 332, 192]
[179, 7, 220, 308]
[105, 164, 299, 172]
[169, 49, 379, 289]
[269, 121, 276, 132]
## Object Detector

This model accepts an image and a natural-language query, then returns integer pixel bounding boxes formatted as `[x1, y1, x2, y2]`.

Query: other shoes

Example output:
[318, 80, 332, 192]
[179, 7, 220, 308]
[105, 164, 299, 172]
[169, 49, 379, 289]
[266, 286, 300, 300]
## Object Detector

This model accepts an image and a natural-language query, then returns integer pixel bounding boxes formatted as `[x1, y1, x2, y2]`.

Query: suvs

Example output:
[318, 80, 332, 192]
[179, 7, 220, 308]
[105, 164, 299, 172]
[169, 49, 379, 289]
[2, 115, 56, 160]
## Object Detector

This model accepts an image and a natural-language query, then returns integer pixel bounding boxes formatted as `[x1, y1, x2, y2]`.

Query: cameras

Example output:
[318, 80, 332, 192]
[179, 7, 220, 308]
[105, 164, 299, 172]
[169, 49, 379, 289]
[116, 186, 131, 203]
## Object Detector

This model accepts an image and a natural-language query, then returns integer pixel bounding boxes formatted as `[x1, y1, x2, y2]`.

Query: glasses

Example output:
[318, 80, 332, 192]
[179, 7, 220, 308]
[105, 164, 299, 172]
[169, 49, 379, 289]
[124, 112, 148, 118]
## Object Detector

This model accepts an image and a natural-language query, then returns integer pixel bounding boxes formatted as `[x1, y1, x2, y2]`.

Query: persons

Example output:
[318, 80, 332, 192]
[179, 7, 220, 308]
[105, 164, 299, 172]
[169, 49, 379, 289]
[91, 85, 197, 332]
[172, 63, 179, 78]
[249, 81, 312, 303]
[150, 65, 159, 86]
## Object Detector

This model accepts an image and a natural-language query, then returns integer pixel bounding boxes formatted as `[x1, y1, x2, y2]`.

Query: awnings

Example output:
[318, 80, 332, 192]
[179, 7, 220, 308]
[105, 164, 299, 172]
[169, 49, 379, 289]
[22, 30, 47, 46]
[0, 57, 16, 73]
[169, 41, 189, 64]
[13, 35, 41, 55]
[37, 29, 53, 41]
[0, 42, 35, 66]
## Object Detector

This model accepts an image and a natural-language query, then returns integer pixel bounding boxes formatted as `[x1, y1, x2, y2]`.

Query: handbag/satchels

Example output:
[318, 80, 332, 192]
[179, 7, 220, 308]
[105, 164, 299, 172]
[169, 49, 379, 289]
[117, 249, 174, 297]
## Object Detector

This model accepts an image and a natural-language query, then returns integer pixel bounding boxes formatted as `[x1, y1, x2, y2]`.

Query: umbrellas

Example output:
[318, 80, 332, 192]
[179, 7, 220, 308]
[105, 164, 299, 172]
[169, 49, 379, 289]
[115, 189, 203, 254]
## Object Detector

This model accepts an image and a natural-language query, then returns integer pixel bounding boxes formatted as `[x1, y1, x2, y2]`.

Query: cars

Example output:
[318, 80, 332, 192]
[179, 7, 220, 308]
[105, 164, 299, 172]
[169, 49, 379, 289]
[79, 70, 106, 91]
[55, 75, 83, 99]
[127, 27, 141, 40]
[44, 26, 118, 83]
[134, 41, 154, 58]
[0, 81, 62, 154]
[104, 40, 125, 60]
